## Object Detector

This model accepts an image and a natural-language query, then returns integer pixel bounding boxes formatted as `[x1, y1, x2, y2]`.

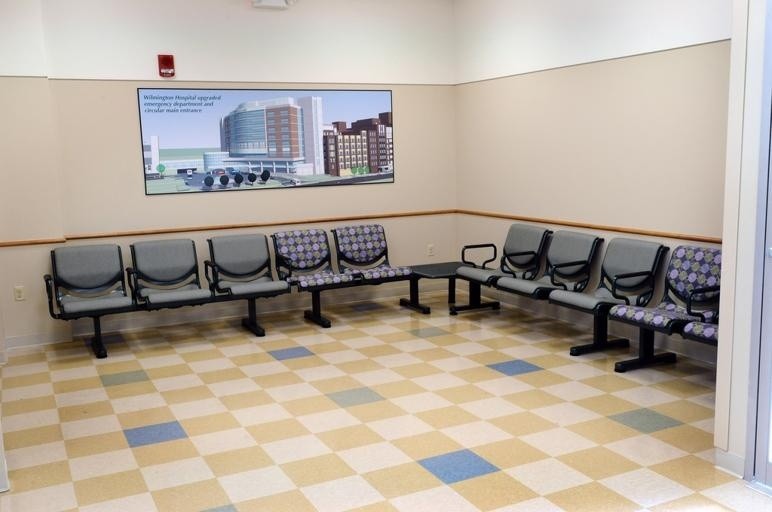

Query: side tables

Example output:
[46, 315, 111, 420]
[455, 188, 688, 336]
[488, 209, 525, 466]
[410, 262, 496, 315]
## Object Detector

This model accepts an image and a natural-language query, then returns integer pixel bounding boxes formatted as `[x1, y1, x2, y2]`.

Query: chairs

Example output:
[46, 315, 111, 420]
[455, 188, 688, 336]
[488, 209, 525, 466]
[331, 224, 430, 314]
[450, 224, 549, 314]
[550, 237, 670, 357]
[609, 245, 720, 373]
[271, 229, 355, 328]
[682, 285, 719, 346]
[206, 234, 292, 337]
[130, 238, 214, 311]
[496, 230, 605, 300]
[43, 233, 139, 358]
